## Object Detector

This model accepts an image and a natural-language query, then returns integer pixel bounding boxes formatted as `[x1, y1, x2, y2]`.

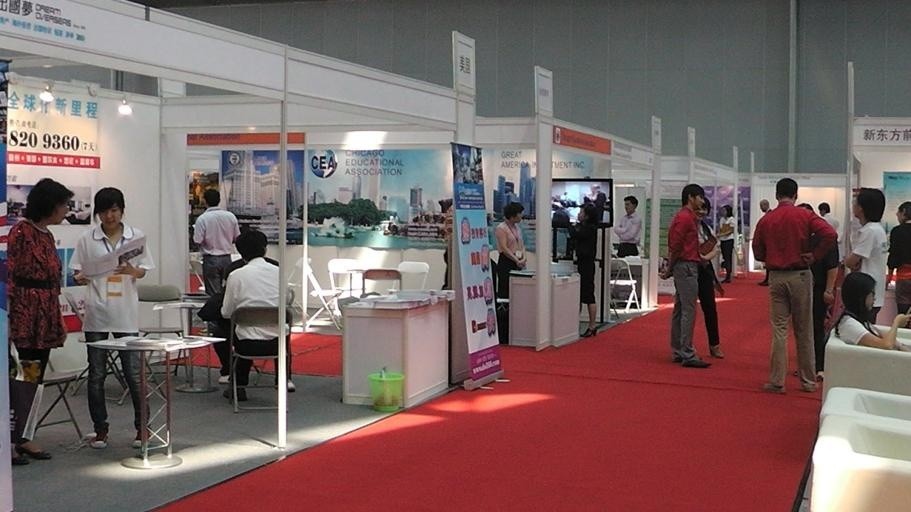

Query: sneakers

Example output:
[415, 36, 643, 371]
[721, 279, 731, 283]
[757, 280, 769, 286]
[763, 383, 786, 394]
[219, 375, 231, 384]
[803, 383, 818, 392]
[223, 386, 249, 402]
[275, 379, 297, 393]
[133, 431, 151, 448]
[91, 433, 107, 449]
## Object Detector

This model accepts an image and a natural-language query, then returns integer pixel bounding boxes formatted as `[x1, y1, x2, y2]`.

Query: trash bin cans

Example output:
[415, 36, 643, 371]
[368, 371, 405, 413]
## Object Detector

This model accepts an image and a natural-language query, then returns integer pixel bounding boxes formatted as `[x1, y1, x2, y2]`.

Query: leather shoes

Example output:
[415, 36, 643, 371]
[710, 349, 725, 359]
[683, 359, 711, 369]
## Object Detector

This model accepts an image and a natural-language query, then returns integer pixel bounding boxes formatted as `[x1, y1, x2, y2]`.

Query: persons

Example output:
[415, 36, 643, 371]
[614, 196, 642, 258]
[198, 231, 296, 392]
[572, 204, 597, 337]
[496, 202, 528, 298]
[752, 178, 838, 392]
[561, 192, 572, 207]
[844, 189, 888, 324]
[7, 178, 74, 466]
[695, 198, 725, 358]
[835, 272, 911, 350]
[193, 188, 241, 296]
[796, 203, 839, 382]
[658, 184, 712, 367]
[221, 231, 291, 400]
[68, 187, 156, 448]
[756, 199, 773, 286]
[583, 185, 606, 222]
[887, 201, 911, 314]
[391, 212, 399, 237]
[717, 205, 736, 284]
[818, 201, 842, 246]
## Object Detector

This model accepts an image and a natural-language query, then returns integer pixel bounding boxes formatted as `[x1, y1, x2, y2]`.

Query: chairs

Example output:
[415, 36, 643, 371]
[609, 259, 641, 318]
[328, 258, 363, 327]
[388, 261, 429, 294]
[65, 292, 131, 394]
[36, 360, 86, 437]
[135, 285, 190, 382]
[362, 269, 401, 296]
[286, 256, 312, 318]
[227, 308, 291, 412]
[297, 257, 342, 330]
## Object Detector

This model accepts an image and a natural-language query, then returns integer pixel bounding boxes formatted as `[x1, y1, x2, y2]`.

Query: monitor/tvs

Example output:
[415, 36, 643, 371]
[551, 179, 614, 228]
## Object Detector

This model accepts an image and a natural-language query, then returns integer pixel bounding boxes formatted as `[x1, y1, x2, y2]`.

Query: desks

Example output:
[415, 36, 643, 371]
[343, 288, 453, 406]
[612, 259, 649, 311]
[155, 302, 210, 392]
[87, 337, 229, 469]
[508, 272, 584, 345]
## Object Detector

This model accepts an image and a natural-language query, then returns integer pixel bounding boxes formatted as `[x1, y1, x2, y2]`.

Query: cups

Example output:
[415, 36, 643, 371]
[430, 290, 442, 303]
[386, 288, 398, 300]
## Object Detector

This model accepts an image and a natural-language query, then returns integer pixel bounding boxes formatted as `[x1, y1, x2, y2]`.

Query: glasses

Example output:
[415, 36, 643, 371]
[869, 289, 876, 296]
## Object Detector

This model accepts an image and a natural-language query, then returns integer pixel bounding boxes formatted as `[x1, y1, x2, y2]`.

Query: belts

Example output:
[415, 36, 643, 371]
[766, 267, 811, 272]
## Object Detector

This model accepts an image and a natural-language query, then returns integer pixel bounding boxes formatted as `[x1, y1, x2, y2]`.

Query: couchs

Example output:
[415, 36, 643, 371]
[819, 323, 909, 405]
[818, 386, 910, 426]
[810, 416, 910, 511]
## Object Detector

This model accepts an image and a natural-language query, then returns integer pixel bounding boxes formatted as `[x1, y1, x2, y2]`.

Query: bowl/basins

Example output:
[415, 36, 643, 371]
[626, 256, 640, 262]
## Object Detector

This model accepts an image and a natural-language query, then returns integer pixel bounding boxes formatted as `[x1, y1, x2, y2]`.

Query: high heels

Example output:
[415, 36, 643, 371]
[14, 446, 52, 461]
[579, 328, 597, 337]
[13, 452, 28, 466]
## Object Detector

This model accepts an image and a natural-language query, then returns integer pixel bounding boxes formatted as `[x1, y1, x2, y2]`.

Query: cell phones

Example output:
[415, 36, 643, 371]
[906, 307, 911, 315]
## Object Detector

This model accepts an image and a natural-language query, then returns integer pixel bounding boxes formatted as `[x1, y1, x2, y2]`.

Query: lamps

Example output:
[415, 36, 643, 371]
[38, 78, 61, 101]
[118, 95, 132, 115]
